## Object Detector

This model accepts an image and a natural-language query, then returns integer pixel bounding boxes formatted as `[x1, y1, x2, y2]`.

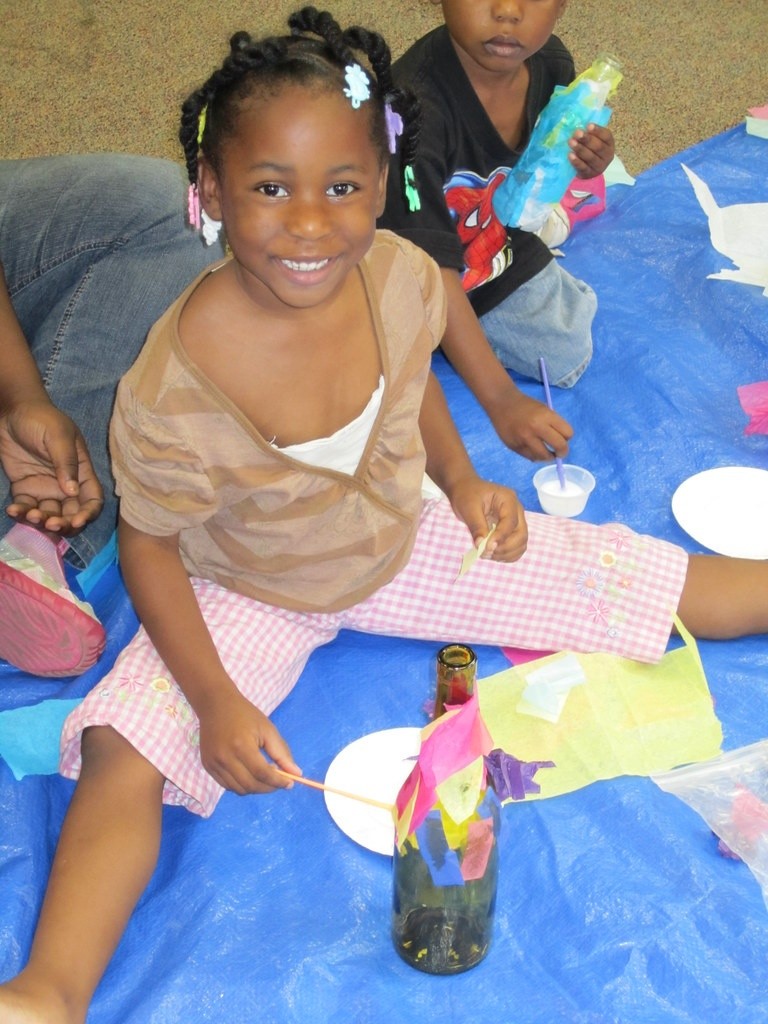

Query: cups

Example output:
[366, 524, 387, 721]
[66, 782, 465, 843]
[532, 464, 595, 519]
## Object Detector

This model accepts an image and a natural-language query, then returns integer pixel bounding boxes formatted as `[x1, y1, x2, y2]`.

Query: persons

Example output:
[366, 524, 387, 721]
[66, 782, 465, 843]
[376, 0, 616, 389]
[0, 155, 222, 677]
[0, 6, 768, 1023]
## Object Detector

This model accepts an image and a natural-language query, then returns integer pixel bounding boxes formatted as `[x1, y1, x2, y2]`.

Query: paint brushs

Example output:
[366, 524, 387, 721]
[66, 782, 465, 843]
[539, 357, 568, 494]
[273, 766, 395, 812]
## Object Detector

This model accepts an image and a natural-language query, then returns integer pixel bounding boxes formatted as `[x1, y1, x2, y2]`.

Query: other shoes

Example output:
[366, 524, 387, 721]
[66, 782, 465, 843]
[0, 524, 105, 679]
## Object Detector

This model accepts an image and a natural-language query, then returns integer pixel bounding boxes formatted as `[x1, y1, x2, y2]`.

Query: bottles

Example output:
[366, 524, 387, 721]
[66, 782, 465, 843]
[394, 642, 498, 973]
[491, 51, 625, 235]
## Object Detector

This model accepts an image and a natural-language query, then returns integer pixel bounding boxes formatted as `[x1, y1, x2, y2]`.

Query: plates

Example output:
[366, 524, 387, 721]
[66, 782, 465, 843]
[324, 727, 426, 857]
[670, 466, 768, 560]
